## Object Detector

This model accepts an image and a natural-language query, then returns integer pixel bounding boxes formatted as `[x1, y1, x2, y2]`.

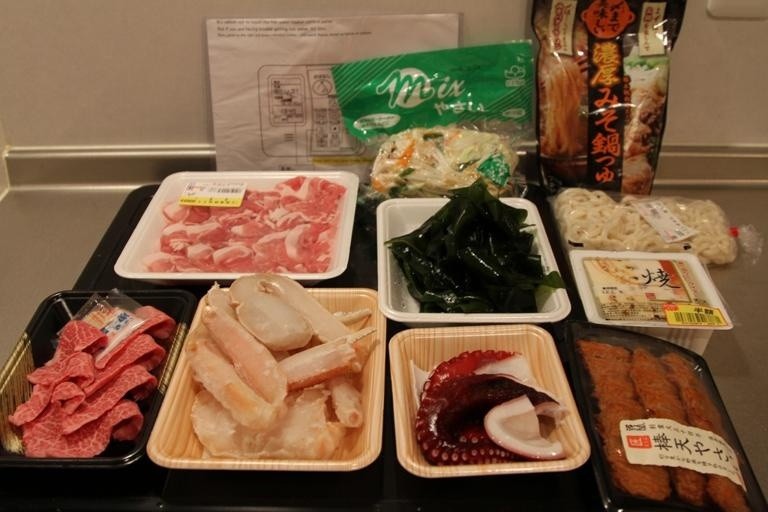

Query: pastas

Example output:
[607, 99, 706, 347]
[543, 59, 584, 156]
[554, 186, 737, 264]
